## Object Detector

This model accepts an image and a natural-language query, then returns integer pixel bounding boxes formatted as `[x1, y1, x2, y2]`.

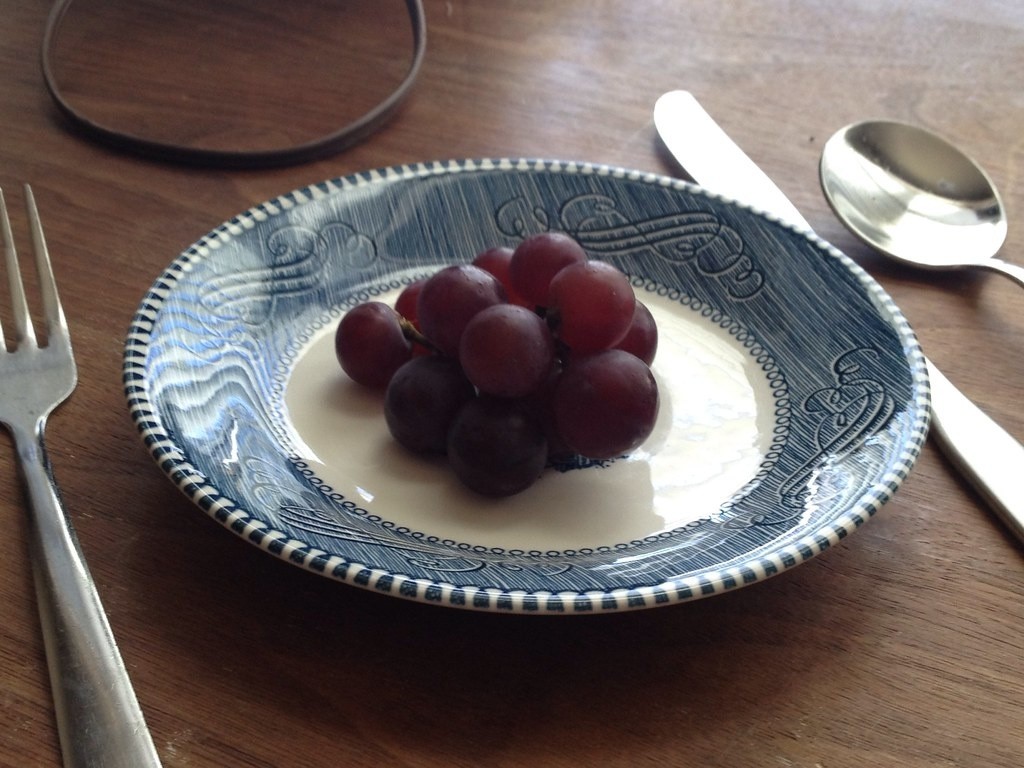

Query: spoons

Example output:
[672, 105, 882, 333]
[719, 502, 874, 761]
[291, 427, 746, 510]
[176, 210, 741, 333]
[819, 119, 1024, 287]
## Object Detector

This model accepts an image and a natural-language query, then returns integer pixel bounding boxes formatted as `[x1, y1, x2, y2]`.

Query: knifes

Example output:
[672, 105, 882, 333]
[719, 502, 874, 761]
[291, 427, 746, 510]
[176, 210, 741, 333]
[653, 89, 1023, 551]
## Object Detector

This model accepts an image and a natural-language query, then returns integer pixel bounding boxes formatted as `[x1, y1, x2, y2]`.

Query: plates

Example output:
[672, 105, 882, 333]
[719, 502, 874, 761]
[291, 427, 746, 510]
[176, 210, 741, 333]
[123, 157, 932, 612]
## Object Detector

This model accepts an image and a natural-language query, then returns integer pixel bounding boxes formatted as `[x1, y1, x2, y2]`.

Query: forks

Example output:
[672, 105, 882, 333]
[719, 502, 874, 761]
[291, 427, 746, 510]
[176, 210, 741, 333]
[0, 180, 158, 768]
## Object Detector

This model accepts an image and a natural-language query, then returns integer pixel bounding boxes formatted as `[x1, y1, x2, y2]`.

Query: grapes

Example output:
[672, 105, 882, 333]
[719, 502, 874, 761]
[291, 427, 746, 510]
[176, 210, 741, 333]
[336, 231, 659, 498]
